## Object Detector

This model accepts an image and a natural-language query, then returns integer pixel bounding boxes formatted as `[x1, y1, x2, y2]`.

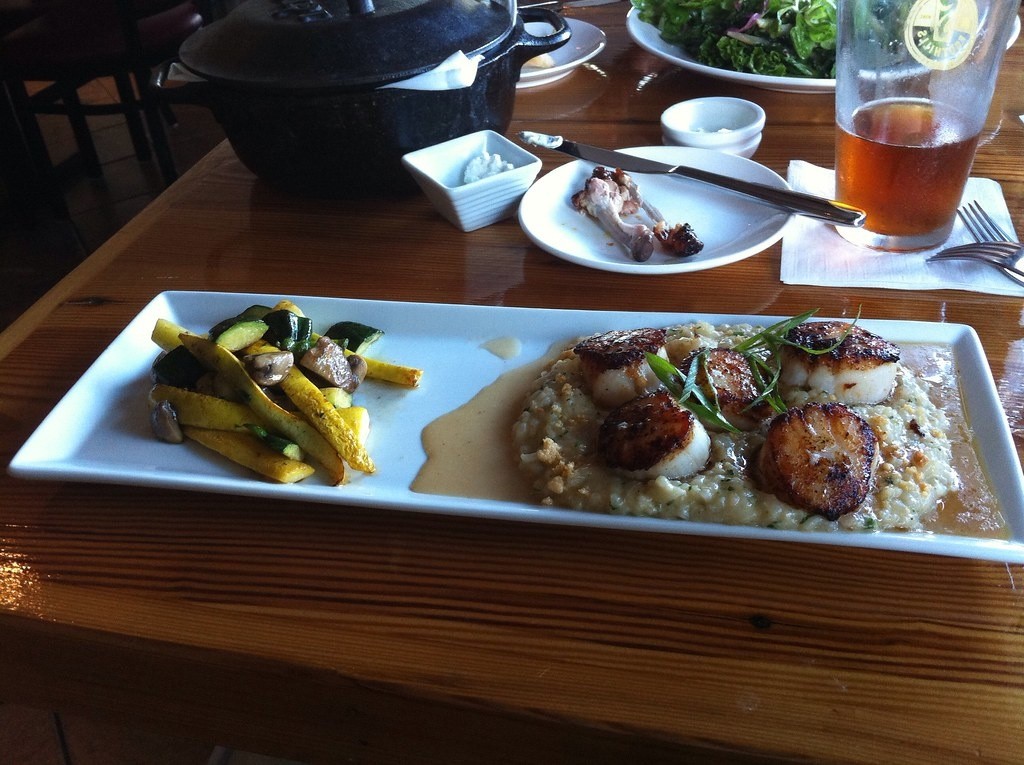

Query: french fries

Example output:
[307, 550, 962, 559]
[148, 300, 424, 487]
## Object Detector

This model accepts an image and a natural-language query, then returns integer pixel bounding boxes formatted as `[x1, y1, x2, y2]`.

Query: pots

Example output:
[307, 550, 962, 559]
[147, 0, 571, 202]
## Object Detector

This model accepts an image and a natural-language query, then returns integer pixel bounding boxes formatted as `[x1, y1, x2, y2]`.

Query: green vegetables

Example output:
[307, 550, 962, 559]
[640, 305, 862, 436]
[630, 0, 944, 78]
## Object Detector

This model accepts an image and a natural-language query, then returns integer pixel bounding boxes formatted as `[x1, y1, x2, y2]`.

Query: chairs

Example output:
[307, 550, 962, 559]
[0, 0, 194, 182]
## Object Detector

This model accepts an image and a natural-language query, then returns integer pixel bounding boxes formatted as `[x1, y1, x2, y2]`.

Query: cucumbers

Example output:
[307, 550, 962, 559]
[324, 321, 385, 354]
[207, 318, 269, 352]
[260, 310, 313, 342]
[265, 434, 307, 462]
[151, 344, 205, 388]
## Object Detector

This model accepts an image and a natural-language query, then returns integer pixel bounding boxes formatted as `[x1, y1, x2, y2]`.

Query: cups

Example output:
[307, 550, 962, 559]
[833, 0, 1023, 254]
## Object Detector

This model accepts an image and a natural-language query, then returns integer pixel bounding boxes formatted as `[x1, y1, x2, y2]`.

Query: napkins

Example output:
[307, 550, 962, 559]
[782, 154, 1023, 299]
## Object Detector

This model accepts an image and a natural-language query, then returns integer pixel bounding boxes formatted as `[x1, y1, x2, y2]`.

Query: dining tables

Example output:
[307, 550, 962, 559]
[0, 0, 1020, 761]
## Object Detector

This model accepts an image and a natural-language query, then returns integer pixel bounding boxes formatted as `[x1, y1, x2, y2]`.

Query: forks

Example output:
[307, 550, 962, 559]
[956, 199, 1024, 288]
[925, 241, 1024, 279]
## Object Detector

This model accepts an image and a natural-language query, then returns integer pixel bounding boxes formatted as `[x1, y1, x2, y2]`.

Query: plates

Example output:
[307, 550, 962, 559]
[518, 144, 795, 275]
[516, 16, 608, 90]
[625, 2, 1020, 94]
[9, 290, 1024, 567]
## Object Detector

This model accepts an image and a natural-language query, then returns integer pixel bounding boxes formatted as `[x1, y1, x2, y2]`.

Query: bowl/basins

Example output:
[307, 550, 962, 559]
[659, 96, 767, 163]
[401, 129, 543, 232]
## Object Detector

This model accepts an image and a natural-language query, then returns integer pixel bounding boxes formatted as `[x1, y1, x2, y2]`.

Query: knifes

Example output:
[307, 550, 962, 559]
[516, 129, 867, 228]
